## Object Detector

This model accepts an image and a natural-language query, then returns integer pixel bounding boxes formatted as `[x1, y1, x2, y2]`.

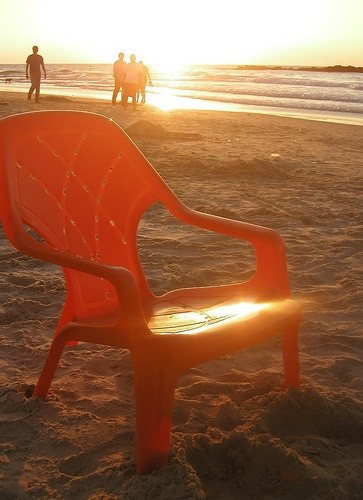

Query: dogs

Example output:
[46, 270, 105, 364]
[6, 78, 13, 83]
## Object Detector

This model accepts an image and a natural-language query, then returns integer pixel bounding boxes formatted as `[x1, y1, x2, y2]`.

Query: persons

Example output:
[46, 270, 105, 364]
[112, 53, 153, 111]
[26, 46, 46, 103]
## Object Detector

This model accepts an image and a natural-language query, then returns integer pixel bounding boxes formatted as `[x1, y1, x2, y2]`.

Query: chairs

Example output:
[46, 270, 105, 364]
[0, 110, 302, 475]
